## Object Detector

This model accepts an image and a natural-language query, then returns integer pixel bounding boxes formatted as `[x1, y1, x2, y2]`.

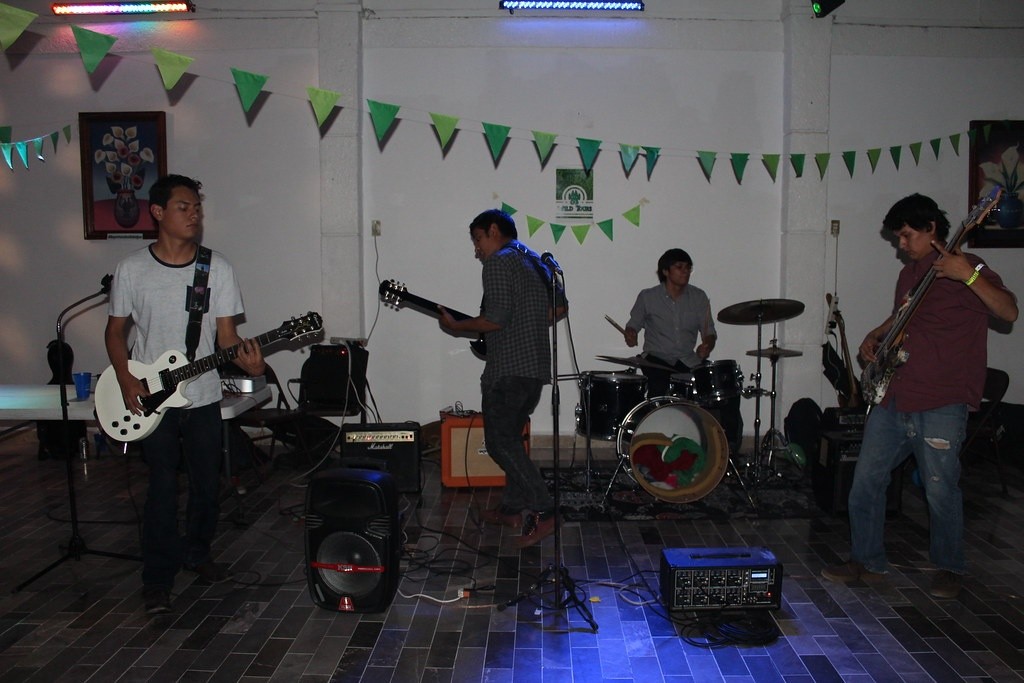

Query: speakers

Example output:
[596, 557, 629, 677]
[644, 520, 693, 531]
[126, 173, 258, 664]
[814, 430, 903, 522]
[298, 346, 369, 412]
[303, 467, 402, 615]
[340, 422, 421, 493]
[441, 414, 530, 487]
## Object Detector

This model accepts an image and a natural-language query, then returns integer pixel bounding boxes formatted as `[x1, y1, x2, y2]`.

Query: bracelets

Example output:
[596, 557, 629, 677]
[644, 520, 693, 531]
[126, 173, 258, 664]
[965, 270, 979, 285]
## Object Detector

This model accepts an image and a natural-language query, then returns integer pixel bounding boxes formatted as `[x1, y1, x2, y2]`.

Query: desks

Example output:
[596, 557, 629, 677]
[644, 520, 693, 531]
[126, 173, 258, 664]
[1, 383, 272, 531]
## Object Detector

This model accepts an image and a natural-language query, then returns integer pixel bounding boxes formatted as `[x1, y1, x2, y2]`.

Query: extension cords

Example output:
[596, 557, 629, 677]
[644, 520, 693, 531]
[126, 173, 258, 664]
[825, 296, 838, 334]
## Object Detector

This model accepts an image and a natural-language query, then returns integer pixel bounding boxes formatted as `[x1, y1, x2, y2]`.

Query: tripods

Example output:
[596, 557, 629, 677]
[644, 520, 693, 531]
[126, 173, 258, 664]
[9, 273, 144, 599]
[546, 366, 636, 496]
[497, 271, 600, 633]
[733, 310, 810, 490]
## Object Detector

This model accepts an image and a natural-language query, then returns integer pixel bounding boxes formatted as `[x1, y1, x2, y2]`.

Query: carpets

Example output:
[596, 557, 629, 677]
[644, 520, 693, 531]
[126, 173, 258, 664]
[539, 463, 828, 522]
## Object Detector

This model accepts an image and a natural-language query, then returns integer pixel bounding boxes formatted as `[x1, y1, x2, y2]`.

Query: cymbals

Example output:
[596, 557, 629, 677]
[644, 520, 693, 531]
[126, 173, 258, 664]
[716, 299, 808, 325]
[593, 354, 673, 372]
[746, 346, 803, 359]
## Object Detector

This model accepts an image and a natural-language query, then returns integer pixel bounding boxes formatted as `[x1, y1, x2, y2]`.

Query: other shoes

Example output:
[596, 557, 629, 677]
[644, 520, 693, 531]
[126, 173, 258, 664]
[479, 504, 523, 529]
[511, 510, 565, 549]
[928, 566, 964, 599]
[183, 559, 234, 584]
[820, 559, 889, 583]
[142, 588, 171, 615]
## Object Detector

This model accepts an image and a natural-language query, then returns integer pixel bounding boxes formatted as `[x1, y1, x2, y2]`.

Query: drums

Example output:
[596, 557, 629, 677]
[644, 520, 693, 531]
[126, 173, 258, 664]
[575, 370, 651, 443]
[667, 358, 744, 406]
[615, 395, 730, 504]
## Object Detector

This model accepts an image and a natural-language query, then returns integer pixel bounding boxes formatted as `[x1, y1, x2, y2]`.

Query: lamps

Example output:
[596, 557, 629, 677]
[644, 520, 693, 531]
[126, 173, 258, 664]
[812, 1, 846, 19]
[499, 0, 646, 11]
[51, 0, 196, 15]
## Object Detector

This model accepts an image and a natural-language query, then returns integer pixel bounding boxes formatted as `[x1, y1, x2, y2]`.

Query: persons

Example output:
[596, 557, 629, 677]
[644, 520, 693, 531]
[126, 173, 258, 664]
[625, 248, 718, 418]
[437, 209, 568, 549]
[104, 174, 265, 614]
[821, 194, 1018, 597]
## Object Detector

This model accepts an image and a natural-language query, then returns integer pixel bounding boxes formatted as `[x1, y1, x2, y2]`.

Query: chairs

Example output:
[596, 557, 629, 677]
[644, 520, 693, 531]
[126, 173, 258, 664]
[218, 345, 368, 486]
[903, 367, 1012, 499]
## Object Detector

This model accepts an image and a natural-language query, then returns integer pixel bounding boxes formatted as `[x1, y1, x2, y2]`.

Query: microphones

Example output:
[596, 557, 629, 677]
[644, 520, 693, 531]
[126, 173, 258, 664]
[541, 252, 563, 275]
[290, 378, 319, 384]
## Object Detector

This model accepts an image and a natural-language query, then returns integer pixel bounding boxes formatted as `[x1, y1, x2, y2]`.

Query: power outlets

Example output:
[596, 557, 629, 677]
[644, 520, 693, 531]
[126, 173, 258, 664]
[372, 220, 381, 236]
[832, 220, 839, 235]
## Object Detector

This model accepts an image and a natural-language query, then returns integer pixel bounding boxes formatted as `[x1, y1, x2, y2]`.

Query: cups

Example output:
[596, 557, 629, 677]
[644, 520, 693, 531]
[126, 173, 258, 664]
[73, 372, 91, 400]
[94, 433, 107, 451]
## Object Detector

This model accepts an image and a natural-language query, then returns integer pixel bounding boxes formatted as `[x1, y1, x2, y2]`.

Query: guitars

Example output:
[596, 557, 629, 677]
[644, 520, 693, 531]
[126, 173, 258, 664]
[378, 278, 489, 359]
[860, 186, 1001, 407]
[94, 309, 324, 442]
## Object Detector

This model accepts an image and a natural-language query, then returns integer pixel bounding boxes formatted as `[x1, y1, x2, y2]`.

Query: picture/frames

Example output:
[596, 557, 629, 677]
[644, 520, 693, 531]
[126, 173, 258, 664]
[969, 120, 1024, 250]
[78, 111, 168, 240]
